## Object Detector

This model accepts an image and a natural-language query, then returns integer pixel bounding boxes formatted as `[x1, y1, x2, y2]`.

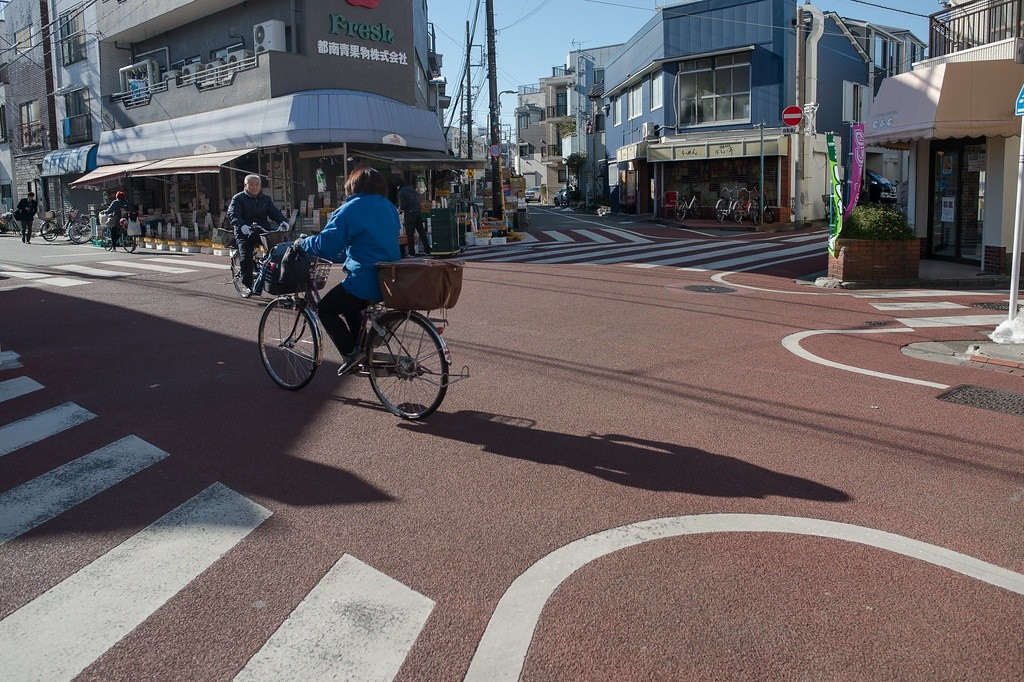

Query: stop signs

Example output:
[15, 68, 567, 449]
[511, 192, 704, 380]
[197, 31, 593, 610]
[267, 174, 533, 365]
[782, 105, 804, 128]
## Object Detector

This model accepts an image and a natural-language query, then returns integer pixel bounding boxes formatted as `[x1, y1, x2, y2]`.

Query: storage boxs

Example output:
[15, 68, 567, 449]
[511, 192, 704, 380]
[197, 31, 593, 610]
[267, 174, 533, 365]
[313, 209, 326, 231]
[374, 258, 464, 310]
[38, 212, 53, 219]
[92, 239, 239, 256]
[431, 169, 525, 251]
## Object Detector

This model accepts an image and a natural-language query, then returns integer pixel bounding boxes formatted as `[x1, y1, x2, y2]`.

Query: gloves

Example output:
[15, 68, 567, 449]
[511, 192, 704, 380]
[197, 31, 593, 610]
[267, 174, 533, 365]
[280, 221, 289, 233]
[240, 225, 252, 236]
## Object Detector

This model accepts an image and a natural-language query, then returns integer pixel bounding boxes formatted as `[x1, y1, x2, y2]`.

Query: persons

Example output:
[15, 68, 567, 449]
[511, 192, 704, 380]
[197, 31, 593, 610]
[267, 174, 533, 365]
[540, 183, 545, 204]
[227, 174, 290, 297]
[103, 191, 129, 252]
[292, 165, 401, 377]
[124, 207, 142, 250]
[398, 177, 432, 258]
[869, 176, 882, 204]
[17, 192, 38, 244]
[388, 182, 407, 258]
[568, 184, 578, 200]
[857, 178, 869, 205]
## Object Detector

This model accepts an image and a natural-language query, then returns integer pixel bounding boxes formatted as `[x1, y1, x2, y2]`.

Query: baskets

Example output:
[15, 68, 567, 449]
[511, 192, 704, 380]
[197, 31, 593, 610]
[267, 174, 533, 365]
[260, 230, 285, 249]
[259, 257, 333, 294]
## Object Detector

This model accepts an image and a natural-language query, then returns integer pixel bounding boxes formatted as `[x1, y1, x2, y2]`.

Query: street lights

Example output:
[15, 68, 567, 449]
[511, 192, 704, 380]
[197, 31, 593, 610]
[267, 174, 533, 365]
[489, 90, 520, 219]
[467, 133, 486, 203]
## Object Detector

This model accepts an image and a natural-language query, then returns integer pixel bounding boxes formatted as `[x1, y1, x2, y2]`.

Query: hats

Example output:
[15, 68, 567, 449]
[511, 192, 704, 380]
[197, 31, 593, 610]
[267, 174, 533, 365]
[115, 191, 124, 199]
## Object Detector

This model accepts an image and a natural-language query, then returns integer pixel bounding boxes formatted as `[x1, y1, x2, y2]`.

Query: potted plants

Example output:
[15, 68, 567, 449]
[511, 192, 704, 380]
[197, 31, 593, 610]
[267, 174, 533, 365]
[828, 201, 919, 281]
[566, 153, 586, 171]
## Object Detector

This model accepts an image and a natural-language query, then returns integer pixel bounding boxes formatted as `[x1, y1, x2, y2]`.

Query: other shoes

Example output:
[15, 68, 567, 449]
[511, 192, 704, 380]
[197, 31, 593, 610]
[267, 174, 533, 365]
[406, 254, 415, 257]
[27, 240, 31, 244]
[21, 234, 25, 243]
[425, 251, 430, 256]
[111, 248, 116, 252]
[241, 284, 252, 294]
[337, 346, 368, 376]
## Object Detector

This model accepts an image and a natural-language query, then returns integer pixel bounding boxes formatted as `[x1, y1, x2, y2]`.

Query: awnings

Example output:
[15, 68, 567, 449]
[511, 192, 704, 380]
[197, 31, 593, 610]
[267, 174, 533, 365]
[68, 148, 305, 187]
[39, 144, 98, 177]
[864, 60, 1024, 150]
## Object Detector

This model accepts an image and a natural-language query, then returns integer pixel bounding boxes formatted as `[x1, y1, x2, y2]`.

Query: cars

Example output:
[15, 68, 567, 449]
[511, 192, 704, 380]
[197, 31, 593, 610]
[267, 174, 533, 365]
[553, 184, 567, 206]
[502, 179, 511, 193]
[524, 185, 541, 203]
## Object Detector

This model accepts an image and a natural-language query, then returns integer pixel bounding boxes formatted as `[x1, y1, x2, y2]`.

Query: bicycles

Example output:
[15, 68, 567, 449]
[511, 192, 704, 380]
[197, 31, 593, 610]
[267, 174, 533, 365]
[714, 184, 775, 227]
[217, 222, 286, 298]
[100, 211, 138, 253]
[37, 204, 92, 244]
[257, 233, 470, 422]
[673, 183, 703, 221]
[0, 212, 21, 235]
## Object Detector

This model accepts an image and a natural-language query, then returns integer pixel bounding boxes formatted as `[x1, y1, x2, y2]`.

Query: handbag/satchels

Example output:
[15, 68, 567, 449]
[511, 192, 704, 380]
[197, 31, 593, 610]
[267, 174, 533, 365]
[13, 210, 21, 221]
[127, 217, 141, 236]
[374, 257, 466, 312]
[252, 241, 310, 293]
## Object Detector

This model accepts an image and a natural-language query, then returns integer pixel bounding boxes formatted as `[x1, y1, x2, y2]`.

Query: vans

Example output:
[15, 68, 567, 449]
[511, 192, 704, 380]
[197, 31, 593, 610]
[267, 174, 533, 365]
[860, 167, 899, 205]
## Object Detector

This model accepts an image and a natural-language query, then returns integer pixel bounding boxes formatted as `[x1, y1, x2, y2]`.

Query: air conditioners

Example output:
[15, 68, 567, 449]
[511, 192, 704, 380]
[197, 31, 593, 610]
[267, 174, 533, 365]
[162, 69, 182, 89]
[205, 61, 225, 80]
[253, 19, 286, 53]
[227, 50, 252, 70]
[181, 63, 205, 84]
[642, 121, 654, 137]
